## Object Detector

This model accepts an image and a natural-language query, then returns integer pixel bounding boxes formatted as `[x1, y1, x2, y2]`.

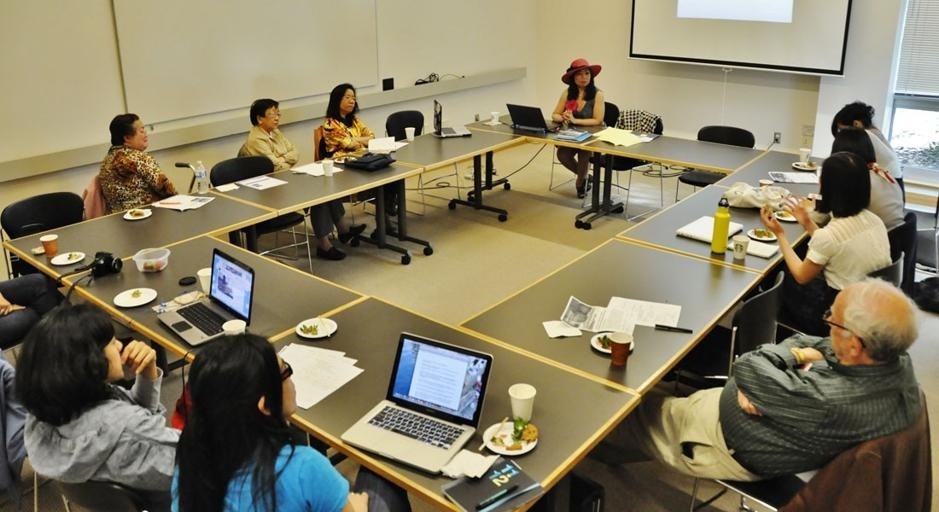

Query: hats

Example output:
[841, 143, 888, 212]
[562, 59, 601, 85]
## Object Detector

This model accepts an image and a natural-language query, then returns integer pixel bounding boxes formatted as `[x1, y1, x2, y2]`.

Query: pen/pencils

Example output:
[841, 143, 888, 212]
[655, 325, 693, 334]
[475, 485, 519, 510]
[160, 202, 179, 204]
[291, 172, 306, 174]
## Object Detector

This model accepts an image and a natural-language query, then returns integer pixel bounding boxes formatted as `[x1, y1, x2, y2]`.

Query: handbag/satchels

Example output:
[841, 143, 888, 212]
[346, 150, 397, 172]
[723, 182, 790, 210]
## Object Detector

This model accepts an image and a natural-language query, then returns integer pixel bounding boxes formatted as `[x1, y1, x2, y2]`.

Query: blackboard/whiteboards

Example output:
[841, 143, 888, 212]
[112, 0, 379, 127]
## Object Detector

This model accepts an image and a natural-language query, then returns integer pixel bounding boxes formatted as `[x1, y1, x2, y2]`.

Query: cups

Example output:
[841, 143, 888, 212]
[759, 180, 773, 187]
[609, 332, 633, 368]
[222, 319, 246, 336]
[800, 148, 809, 162]
[405, 127, 415, 141]
[197, 268, 212, 295]
[732, 235, 750, 260]
[322, 160, 334, 176]
[491, 112, 500, 123]
[40, 234, 58, 258]
[508, 383, 536, 422]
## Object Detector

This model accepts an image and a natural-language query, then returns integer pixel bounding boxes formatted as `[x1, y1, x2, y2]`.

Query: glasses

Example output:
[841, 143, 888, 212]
[268, 112, 281, 117]
[281, 360, 293, 379]
[822, 309, 866, 348]
[343, 95, 356, 100]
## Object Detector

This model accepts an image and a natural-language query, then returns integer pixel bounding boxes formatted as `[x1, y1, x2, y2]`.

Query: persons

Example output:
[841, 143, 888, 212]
[820, 127, 907, 288]
[18, 299, 187, 493]
[96, 111, 180, 216]
[549, 58, 605, 200]
[323, 81, 401, 237]
[240, 97, 367, 260]
[759, 151, 893, 324]
[829, 100, 907, 205]
[0, 268, 64, 356]
[603, 275, 927, 484]
[169, 332, 367, 512]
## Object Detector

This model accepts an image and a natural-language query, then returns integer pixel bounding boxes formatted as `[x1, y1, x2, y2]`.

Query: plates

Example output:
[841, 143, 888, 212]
[113, 288, 157, 308]
[792, 162, 817, 171]
[747, 229, 778, 241]
[50, 252, 86, 265]
[123, 209, 153, 220]
[335, 157, 356, 164]
[773, 210, 797, 222]
[482, 422, 538, 456]
[590, 332, 635, 353]
[296, 318, 337, 338]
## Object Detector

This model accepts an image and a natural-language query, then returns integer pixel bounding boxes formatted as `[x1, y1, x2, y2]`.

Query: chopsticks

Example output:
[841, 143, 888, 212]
[478, 416, 509, 452]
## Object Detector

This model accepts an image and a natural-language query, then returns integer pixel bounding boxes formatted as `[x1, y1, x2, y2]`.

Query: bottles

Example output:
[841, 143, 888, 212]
[194, 160, 208, 194]
[711, 196, 731, 254]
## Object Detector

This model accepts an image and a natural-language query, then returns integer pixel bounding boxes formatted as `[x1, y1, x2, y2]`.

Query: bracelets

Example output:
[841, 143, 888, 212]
[791, 346, 808, 363]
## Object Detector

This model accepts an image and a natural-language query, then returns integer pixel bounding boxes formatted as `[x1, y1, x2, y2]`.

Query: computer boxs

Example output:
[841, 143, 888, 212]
[533, 471, 604, 511]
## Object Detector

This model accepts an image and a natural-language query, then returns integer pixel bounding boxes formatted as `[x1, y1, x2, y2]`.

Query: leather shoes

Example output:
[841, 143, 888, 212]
[317, 245, 345, 259]
[338, 224, 366, 244]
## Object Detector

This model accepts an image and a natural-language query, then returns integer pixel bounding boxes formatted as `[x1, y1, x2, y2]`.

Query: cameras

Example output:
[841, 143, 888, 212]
[92, 252, 123, 276]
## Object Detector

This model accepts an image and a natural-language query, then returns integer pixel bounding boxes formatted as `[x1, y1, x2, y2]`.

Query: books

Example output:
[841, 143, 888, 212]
[552, 126, 592, 143]
[443, 457, 539, 511]
[678, 216, 742, 244]
[727, 236, 781, 259]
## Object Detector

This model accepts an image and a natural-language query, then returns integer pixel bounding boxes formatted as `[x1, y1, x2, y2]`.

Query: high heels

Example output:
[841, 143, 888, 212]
[587, 174, 593, 191]
[576, 178, 586, 198]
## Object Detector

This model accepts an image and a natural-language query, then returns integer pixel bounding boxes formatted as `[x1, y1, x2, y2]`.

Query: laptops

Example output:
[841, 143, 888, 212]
[507, 104, 563, 133]
[157, 248, 255, 347]
[341, 332, 494, 475]
[434, 100, 472, 138]
